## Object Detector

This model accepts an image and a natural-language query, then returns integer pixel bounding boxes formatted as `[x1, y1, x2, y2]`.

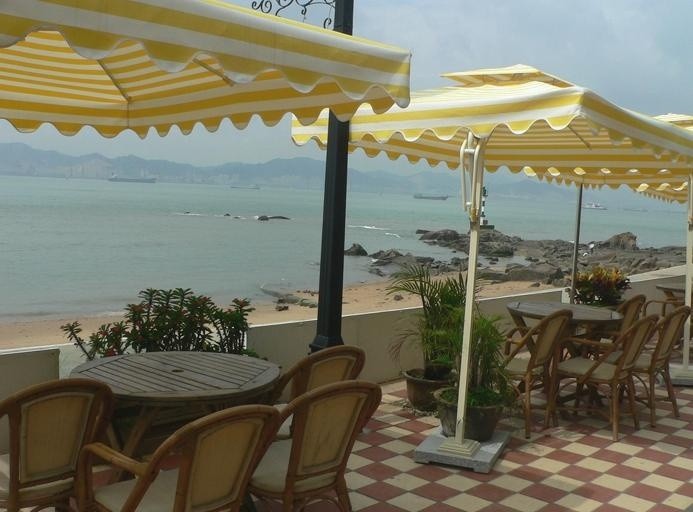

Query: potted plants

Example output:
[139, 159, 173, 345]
[384, 259, 518, 442]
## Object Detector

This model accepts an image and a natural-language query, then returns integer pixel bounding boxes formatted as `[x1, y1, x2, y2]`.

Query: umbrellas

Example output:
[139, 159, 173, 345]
[0, 0, 414, 140]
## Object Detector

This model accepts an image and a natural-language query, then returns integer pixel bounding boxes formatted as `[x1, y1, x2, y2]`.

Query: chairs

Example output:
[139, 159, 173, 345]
[1, 377, 115, 509]
[75, 405, 281, 511]
[240, 379, 383, 511]
[504, 294, 691, 439]
[257, 348, 367, 439]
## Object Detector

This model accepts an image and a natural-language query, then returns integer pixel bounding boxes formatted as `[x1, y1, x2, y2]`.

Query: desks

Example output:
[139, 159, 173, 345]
[656, 283, 686, 308]
[70, 350, 281, 482]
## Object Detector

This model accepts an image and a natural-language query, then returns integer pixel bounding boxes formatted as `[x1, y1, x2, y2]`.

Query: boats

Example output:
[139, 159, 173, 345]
[581, 202, 609, 209]
[232, 185, 259, 189]
[415, 194, 448, 201]
[108, 176, 156, 184]
